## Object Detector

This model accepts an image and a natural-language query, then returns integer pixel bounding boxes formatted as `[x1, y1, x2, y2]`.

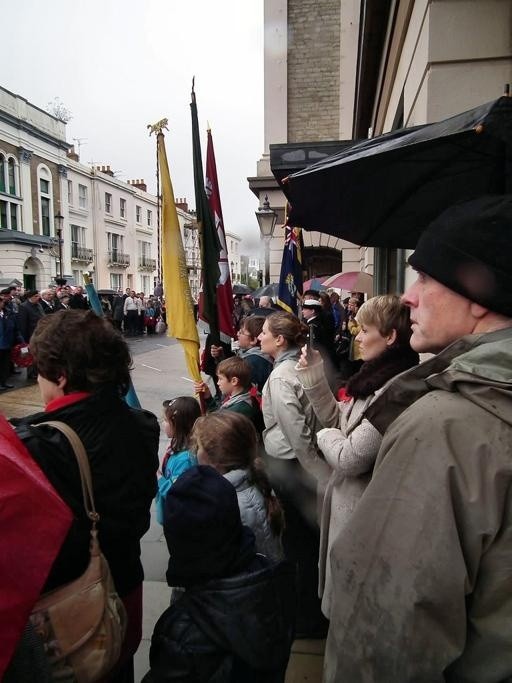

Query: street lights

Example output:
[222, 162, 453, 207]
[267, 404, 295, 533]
[253, 191, 279, 286]
[53, 209, 65, 288]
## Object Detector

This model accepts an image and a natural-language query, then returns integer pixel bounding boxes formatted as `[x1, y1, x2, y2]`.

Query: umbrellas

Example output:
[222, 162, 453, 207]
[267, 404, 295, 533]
[280, 83, 512, 249]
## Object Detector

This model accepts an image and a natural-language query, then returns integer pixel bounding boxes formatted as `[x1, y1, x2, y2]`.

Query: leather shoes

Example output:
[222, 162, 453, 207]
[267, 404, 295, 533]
[3, 383, 14, 388]
[0, 386, 6, 390]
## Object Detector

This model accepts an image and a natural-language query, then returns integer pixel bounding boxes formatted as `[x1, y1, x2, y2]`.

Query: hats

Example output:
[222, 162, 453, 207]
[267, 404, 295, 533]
[302, 299, 322, 310]
[408, 192, 512, 318]
[59, 290, 71, 298]
[163, 464, 255, 588]
[1, 287, 16, 293]
[28, 290, 38, 297]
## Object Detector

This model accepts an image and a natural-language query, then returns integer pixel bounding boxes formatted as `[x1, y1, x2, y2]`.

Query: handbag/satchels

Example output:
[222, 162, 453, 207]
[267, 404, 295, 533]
[3, 551, 129, 682]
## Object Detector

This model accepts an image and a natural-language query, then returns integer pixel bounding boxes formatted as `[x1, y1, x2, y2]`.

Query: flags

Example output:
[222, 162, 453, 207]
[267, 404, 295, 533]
[189, 92, 225, 356]
[150, 130, 209, 395]
[192, 136, 240, 340]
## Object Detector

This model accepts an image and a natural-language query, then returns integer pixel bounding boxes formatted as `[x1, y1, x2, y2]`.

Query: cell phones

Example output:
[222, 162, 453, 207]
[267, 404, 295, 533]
[306, 324, 315, 363]
